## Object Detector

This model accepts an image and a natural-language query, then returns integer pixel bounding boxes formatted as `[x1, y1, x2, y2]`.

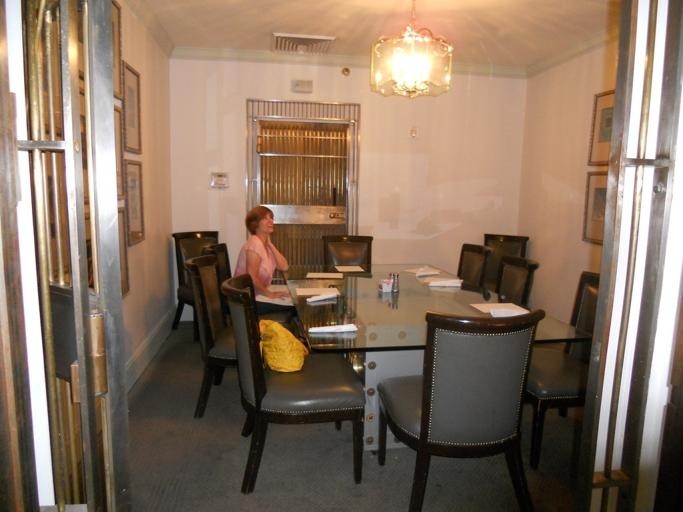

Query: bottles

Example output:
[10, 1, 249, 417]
[393, 273, 399, 292]
[388, 273, 394, 291]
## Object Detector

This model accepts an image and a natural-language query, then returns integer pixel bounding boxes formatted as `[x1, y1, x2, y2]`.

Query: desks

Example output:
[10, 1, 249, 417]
[281, 264, 592, 453]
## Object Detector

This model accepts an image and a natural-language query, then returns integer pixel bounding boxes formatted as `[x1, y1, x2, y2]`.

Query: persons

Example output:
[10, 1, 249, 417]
[233, 205, 308, 348]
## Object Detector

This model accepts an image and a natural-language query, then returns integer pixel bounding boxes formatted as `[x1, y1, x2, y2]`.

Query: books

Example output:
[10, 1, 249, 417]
[421, 275, 464, 287]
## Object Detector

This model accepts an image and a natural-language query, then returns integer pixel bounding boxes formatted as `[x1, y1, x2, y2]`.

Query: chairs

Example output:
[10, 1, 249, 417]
[564, 271, 600, 326]
[494, 255, 539, 306]
[457, 243, 490, 292]
[525, 284, 599, 472]
[184, 254, 237, 418]
[172, 231, 218, 342]
[376, 309, 545, 512]
[201, 243, 233, 315]
[221, 273, 366, 494]
[322, 235, 373, 265]
[484, 233, 529, 292]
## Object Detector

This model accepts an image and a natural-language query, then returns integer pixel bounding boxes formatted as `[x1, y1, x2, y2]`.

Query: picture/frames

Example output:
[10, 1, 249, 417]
[587, 89, 615, 166]
[114, 104, 124, 199]
[118, 207, 130, 299]
[123, 159, 145, 247]
[121, 59, 141, 154]
[582, 171, 608, 247]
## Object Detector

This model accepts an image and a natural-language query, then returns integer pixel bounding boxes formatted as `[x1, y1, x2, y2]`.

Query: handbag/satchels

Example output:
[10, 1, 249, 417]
[259, 319, 309, 373]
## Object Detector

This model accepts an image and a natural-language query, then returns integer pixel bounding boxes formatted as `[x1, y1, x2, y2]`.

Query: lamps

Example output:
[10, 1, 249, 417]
[370, 1, 453, 99]
